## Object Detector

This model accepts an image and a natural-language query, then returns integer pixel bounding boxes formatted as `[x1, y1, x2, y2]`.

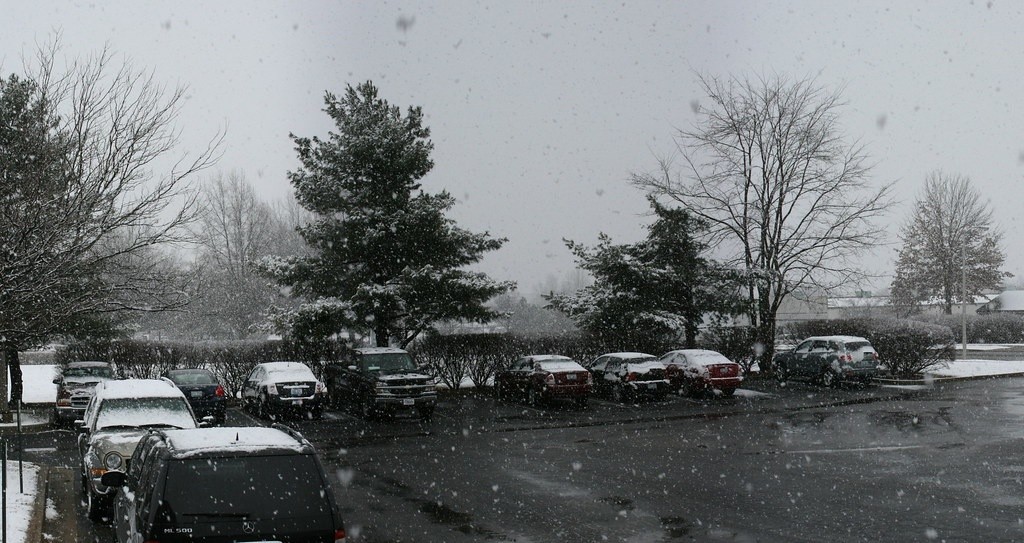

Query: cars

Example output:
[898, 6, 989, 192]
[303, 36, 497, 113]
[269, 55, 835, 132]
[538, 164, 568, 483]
[585, 351, 670, 402]
[241, 360, 328, 421]
[79, 422, 188, 521]
[162, 365, 228, 422]
[493, 354, 593, 408]
[658, 348, 742, 396]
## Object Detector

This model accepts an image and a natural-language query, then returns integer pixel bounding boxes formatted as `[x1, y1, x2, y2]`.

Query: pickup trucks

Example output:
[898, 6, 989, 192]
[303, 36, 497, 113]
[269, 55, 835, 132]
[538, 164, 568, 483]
[325, 345, 438, 422]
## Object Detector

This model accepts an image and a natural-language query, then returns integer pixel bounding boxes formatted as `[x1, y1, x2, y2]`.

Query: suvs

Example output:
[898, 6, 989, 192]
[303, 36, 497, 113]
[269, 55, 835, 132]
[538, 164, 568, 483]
[73, 374, 216, 459]
[101, 421, 348, 543]
[773, 335, 878, 391]
[53, 361, 125, 423]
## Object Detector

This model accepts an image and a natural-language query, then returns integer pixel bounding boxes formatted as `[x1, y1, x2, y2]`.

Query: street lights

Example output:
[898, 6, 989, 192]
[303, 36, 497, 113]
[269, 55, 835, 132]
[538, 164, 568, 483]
[961, 225, 991, 360]
